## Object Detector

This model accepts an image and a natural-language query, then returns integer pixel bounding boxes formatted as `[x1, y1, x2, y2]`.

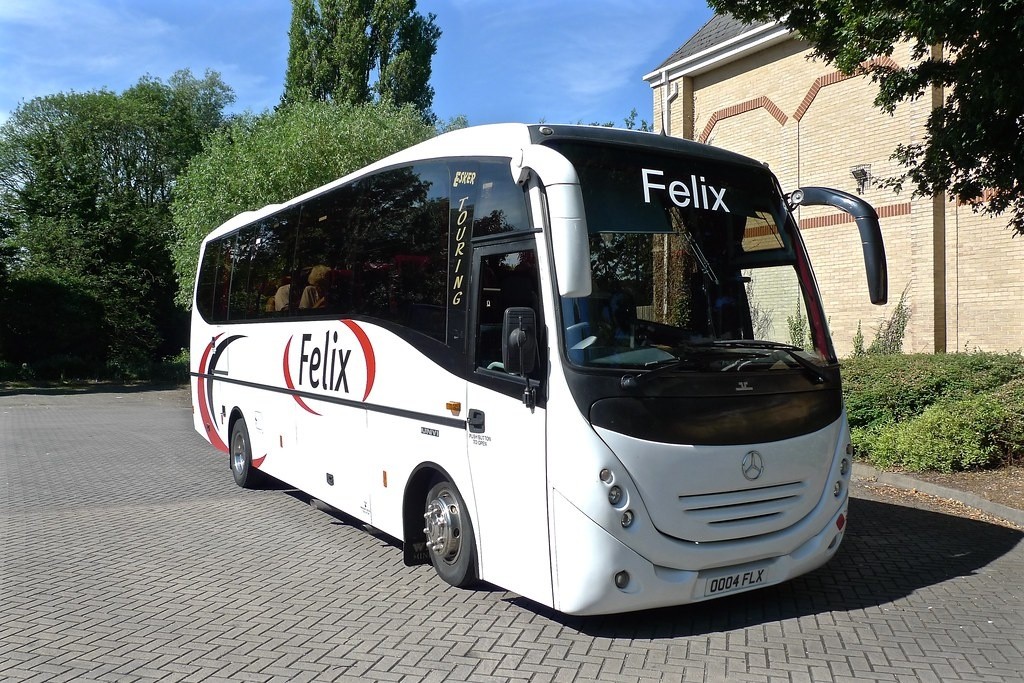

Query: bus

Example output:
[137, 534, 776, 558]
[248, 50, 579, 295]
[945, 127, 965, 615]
[188, 117, 891, 623]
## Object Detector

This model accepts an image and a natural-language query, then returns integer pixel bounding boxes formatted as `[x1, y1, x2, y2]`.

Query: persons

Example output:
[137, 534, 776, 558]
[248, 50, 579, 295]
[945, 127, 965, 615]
[298, 265, 332, 308]
[274, 266, 293, 312]
